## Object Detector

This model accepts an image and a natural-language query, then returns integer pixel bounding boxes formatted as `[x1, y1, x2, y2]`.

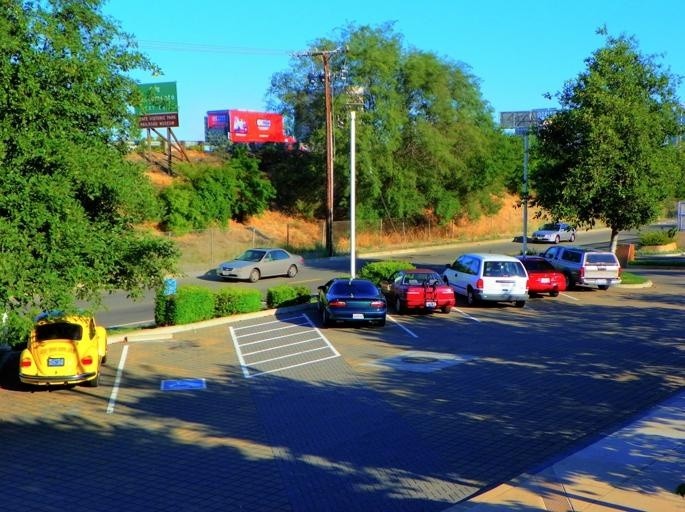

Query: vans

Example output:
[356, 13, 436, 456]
[540, 246, 622, 290]
[442, 252, 530, 307]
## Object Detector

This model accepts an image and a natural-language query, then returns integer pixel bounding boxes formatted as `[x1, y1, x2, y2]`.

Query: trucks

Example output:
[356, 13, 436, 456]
[202, 109, 309, 153]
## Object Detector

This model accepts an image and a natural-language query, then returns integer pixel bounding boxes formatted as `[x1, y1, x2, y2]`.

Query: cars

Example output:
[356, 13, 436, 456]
[531, 223, 576, 244]
[318, 278, 387, 326]
[216, 248, 303, 282]
[378, 269, 455, 313]
[19, 309, 108, 387]
[514, 255, 567, 296]
